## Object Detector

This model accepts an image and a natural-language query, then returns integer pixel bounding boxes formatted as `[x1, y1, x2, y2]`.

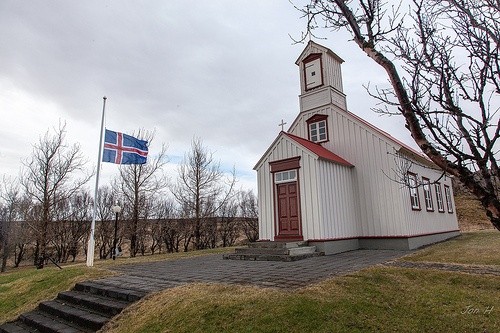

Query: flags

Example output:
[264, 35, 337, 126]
[101, 128, 149, 165]
